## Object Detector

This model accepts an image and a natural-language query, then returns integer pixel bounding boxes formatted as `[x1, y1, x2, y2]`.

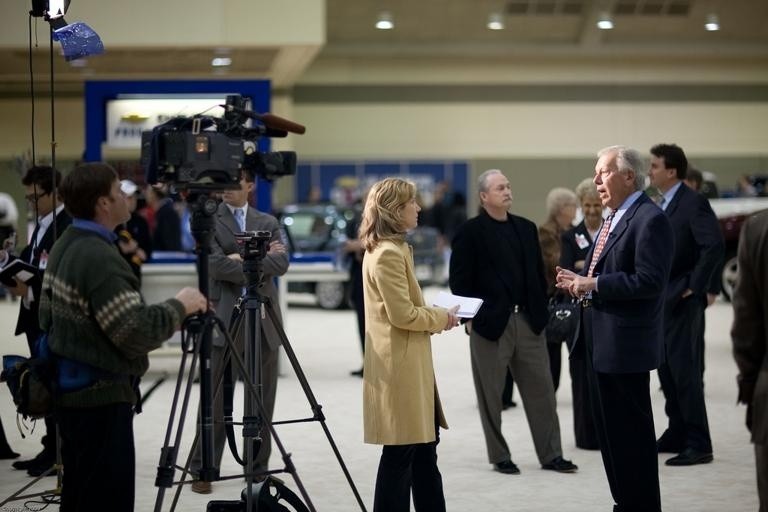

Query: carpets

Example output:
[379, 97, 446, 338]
[234, 208, 245, 232]
[658, 198, 666, 209]
[587, 207, 618, 277]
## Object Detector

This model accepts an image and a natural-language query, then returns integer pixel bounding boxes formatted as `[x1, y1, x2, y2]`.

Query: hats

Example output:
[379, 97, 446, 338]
[270, 203, 439, 309]
[697, 173, 768, 301]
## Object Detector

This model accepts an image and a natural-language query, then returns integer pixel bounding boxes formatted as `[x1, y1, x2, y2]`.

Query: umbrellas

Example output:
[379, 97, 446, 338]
[11, 456, 48, 470]
[493, 459, 520, 475]
[541, 456, 578, 472]
[664, 452, 714, 466]
[26, 464, 57, 477]
[192, 479, 212, 494]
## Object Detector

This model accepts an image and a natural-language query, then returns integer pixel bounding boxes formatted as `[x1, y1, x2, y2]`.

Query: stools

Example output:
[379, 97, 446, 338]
[581, 299, 592, 307]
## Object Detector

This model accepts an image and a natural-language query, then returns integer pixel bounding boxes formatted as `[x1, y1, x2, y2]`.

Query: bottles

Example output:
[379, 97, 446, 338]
[120, 179, 139, 197]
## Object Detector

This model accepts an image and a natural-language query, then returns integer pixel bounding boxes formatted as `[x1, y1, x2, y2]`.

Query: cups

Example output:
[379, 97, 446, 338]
[24, 191, 47, 200]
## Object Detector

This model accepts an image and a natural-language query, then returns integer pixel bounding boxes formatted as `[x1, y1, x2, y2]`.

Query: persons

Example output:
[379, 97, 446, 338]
[448, 167, 579, 474]
[12, 165, 70, 473]
[731, 211, 767, 511]
[538, 178, 606, 450]
[120, 179, 194, 261]
[189, 170, 293, 493]
[646, 144, 727, 469]
[1, 193, 18, 257]
[342, 202, 367, 377]
[38, 158, 218, 511]
[357, 177, 465, 511]
[686, 169, 768, 305]
[554, 145, 677, 511]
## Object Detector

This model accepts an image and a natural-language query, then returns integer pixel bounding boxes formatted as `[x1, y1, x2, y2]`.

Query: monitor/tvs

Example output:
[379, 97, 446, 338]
[0, 258, 44, 289]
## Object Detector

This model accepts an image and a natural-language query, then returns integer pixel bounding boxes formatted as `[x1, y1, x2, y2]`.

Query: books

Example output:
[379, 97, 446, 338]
[0, 258, 44, 289]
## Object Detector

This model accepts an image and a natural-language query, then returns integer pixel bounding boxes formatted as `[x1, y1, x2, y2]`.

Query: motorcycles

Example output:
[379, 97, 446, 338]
[1, 26, 69, 510]
[154, 207, 314, 512]
[167, 230, 368, 512]
